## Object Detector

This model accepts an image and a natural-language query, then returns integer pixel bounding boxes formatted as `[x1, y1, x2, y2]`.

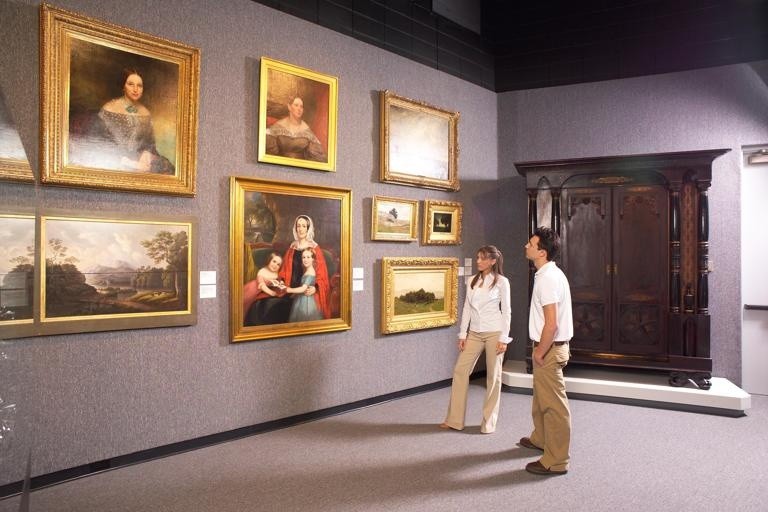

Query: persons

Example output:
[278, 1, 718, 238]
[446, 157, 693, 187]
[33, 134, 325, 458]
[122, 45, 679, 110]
[520, 226, 574, 475]
[244, 215, 331, 326]
[267, 97, 328, 162]
[241, 252, 282, 307]
[442, 244, 511, 434]
[90, 70, 175, 175]
[276, 248, 322, 323]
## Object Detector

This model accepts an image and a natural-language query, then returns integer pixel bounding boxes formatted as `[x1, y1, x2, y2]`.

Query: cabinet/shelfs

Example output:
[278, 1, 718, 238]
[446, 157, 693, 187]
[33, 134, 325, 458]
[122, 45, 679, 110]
[513, 149, 731, 390]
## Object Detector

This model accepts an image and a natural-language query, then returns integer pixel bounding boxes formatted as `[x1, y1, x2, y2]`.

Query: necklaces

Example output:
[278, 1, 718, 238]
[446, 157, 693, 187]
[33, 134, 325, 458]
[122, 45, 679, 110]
[289, 118, 301, 135]
[120, 96, 139, 113]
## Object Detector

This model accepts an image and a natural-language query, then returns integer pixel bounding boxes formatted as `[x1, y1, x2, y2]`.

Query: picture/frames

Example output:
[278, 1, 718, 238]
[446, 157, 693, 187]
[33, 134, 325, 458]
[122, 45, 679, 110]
[371, 194, 419, 242]
[0, 91, 35, 184]
[422, 199, 463, 246]
[0, 206, 200, 339]
[258, 56, 338, 172]
[380, 257, 459, 335]
[379, 89, 460, 192]
[229, 175, 351, 343]
[39, 3, 201, 197]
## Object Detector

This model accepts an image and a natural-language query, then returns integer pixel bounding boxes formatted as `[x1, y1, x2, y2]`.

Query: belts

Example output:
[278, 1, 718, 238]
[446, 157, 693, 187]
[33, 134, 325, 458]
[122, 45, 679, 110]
[534, 342, 564, 347]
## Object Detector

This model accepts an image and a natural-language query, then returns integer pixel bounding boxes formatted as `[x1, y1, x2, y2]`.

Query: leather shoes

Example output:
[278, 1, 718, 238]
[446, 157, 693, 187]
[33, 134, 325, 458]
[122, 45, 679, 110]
[526, 461, 568, 475]
[520, 437, 544, 451]
[440, 424, 451, 429]
